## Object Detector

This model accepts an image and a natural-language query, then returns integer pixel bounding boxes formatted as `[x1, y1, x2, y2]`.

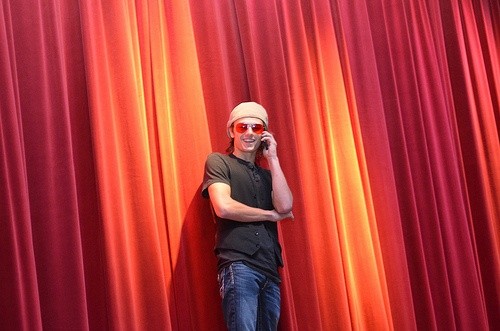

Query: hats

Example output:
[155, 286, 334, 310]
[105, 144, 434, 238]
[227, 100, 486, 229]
[227, 101, 268, 128]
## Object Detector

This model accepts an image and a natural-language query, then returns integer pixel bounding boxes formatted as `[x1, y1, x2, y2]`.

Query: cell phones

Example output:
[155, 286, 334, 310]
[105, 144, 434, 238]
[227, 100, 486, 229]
[264, 139, 268, 150]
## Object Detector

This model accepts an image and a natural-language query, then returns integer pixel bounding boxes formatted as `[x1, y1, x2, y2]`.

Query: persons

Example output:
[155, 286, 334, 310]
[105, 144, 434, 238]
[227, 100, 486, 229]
[201, 102, 294, 331]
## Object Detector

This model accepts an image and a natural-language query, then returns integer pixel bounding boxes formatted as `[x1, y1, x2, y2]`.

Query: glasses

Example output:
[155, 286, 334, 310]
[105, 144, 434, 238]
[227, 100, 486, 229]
[235, 123, 264, 134]
